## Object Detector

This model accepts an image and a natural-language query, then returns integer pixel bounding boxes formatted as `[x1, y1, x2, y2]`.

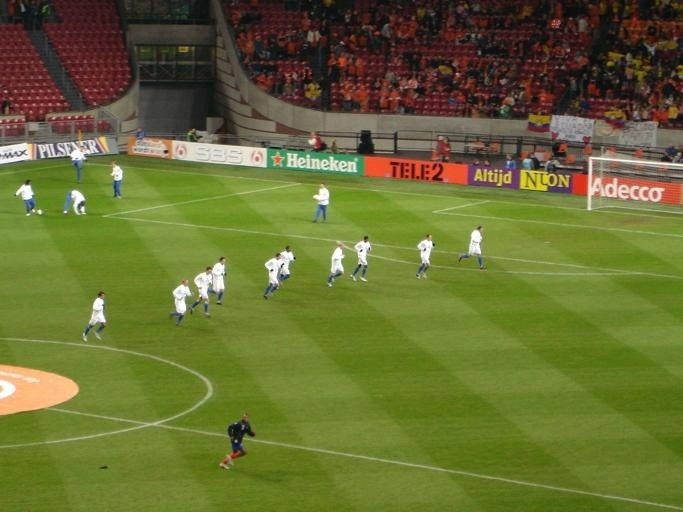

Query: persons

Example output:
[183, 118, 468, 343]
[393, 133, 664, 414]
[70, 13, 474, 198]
[70, 145, 87, 182]
[357, 134, 373, 153]
[279, 245, 295, 281]
[350, 236, 372, 283]
[432, 135, 451, 162]
[217, 412, 255, 470]
[505, 152, 556, 170]
[226, 1, 683, 127]
[63, 189, 87, 215]
[327, 240, 346, 287]
[308, 130, 323, 152]
[110, 160, 123, 198]
[458, 226, 487, 269]
[416, 234, 435, 279]
[330, 139, 337, 154]
[263, 252, 282, 299]
[135, 126, 144, 139]
[552, 137, 568, 157]
[170, 256, 227, 327]
[583, 140, 645, 170]
[313, 183, 330, 223]
[82, 291, 106, 343]
[661, 144, 682, 164]
[186, 128, 198, 142]
[15, 179, 36, 216]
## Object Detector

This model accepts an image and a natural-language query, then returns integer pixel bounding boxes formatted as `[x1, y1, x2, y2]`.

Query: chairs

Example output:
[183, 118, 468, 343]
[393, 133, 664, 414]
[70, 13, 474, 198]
[472, 141, 645, 170]
[0, 0, 132, 137]
[222, 0, 683, 129]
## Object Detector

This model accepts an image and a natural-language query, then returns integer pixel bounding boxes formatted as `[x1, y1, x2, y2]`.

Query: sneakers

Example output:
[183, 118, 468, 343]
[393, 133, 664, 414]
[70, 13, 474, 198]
[169, 301, 222, 326]
[327, 278, 335, 288]
[458, 255, 463, 262]
[219, 455, 234, 469]
[359, 276, 367, 281]
[417, 271, 427, 280]
[350, 274, 357, 282]
[83, 333, 87, 342]
[95, 331, 101, 341]
[481, 266, 487, 270]
[26, 209, 35, 216]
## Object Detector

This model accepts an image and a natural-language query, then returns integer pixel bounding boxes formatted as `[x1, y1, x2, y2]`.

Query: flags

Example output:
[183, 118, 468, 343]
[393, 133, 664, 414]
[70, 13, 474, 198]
[616, 122, 658, 148]
[527, 114, 549, 133]
[595, 120, 619, 137]
[548, 115, 595, 144]
